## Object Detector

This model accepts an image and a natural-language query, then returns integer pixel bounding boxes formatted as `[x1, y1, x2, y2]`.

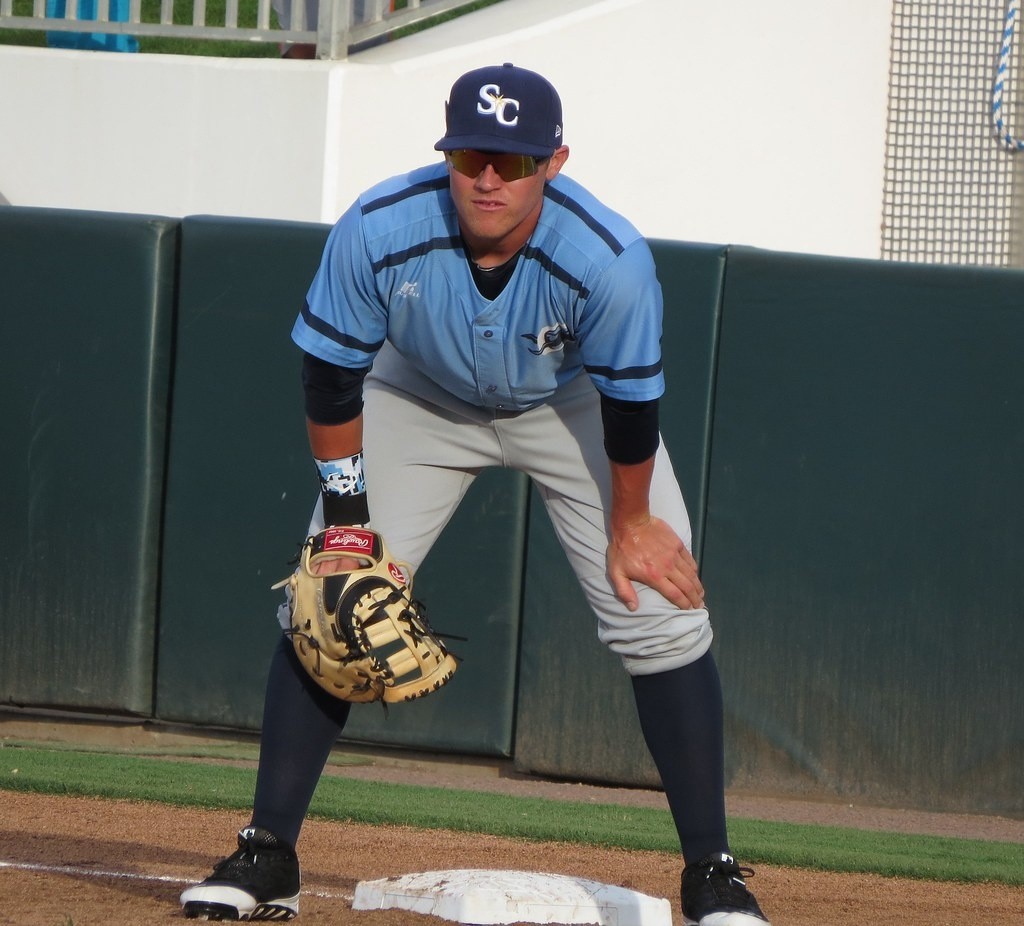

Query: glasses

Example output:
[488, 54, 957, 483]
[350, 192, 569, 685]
[449, 149, 553, 182]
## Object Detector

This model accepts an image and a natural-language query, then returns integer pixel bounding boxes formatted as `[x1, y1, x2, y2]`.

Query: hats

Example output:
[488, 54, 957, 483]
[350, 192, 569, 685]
[434, 63, 563, 158]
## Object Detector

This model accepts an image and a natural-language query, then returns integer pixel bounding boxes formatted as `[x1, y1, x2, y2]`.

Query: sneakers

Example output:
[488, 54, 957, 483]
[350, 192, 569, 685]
[180, 825, 301, 922]
[681, 852, 771, 926]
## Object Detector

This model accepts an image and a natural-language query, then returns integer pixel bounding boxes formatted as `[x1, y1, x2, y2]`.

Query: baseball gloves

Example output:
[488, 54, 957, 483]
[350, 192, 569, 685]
[271, 526, 460, 708]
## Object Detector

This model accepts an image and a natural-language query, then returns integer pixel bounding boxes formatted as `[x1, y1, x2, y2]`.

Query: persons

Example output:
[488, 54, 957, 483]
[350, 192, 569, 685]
[176, 64, 771, 925]
